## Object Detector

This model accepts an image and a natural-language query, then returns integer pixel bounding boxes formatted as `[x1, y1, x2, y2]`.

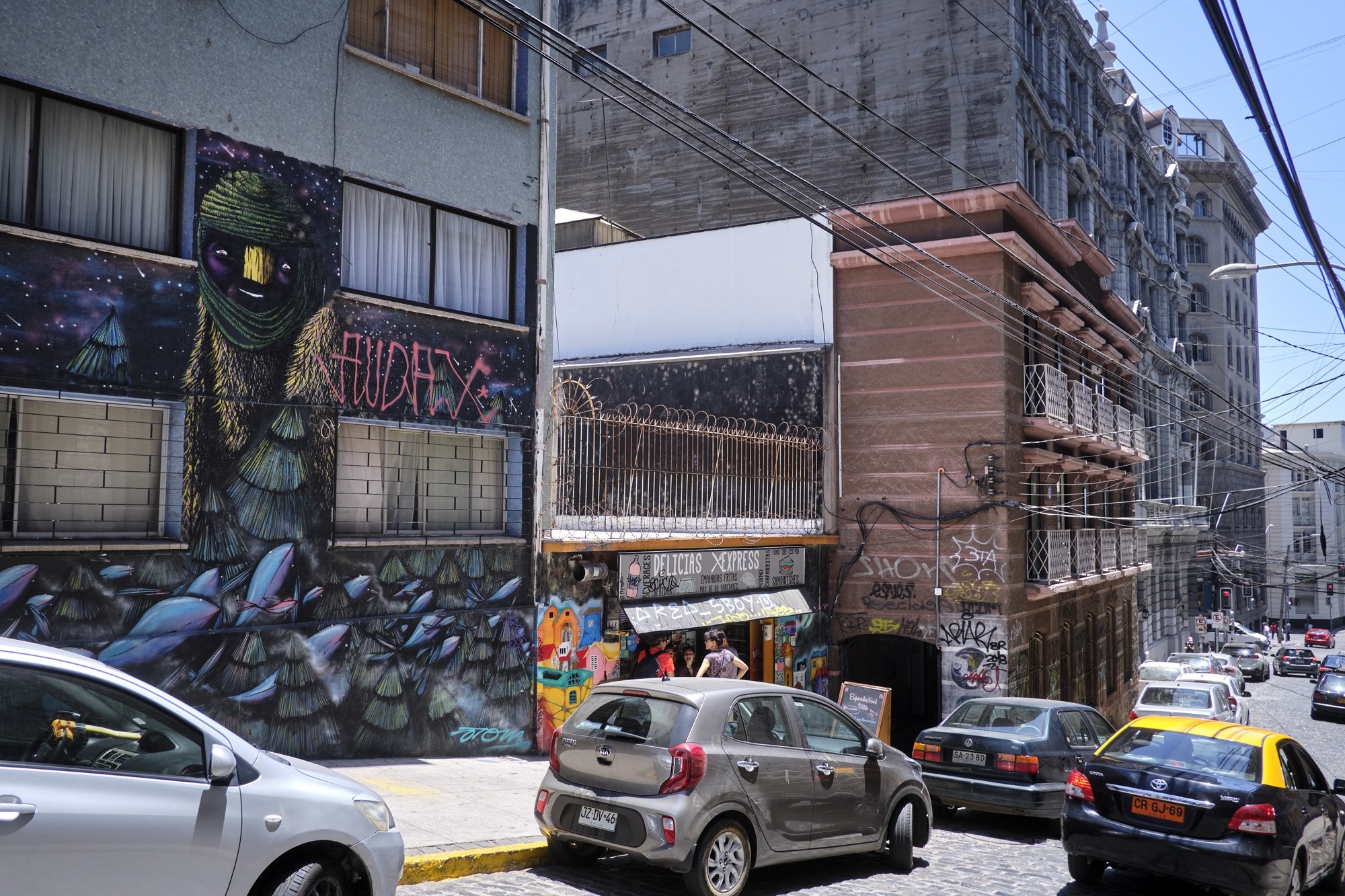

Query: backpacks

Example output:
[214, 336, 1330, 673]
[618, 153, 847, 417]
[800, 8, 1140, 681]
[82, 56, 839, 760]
[634, 647, 666, 678]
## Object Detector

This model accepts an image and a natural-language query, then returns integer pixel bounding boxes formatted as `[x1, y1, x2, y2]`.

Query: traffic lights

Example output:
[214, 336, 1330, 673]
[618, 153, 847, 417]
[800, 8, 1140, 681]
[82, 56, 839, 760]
[1327, 582, 1334, 595]
[1338, 563, 1345, 578]
[1242, 579, 1254, 597]
[1219, 587, 1233, 612]
[1289, 597, 1295, 606]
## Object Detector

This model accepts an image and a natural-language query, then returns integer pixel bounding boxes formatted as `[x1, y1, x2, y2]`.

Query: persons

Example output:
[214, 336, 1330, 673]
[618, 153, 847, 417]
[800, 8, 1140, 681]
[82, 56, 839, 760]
[1198, 637, 1211, 653]
[1184, 637, 1195, 653]
[635, 629, 749, 679]
[1262, 622, 1273, 650]
[1270, 620, 1290, 641]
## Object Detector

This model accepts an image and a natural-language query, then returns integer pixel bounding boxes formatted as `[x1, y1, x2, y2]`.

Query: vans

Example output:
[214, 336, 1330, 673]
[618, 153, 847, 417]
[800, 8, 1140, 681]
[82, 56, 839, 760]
[1199, 618, 1271, 652]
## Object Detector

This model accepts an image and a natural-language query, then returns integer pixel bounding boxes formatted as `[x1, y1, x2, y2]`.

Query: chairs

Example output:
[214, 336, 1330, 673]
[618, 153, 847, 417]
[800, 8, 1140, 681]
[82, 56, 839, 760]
[733, 706, 780, 746]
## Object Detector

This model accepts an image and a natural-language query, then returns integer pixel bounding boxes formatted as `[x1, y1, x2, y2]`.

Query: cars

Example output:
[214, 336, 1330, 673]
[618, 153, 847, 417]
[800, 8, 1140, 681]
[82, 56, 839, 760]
[1315, 655, 1345, 681]
[534, 676, 937, 896]
[1307, 673, 1345, 722]
[1270, 646, 1320, 679]
[1137, 651, 1252, 726]
[1304, 628, 1336, 649]
[911, 695, 1125, 821]
[1061, 714, 1345, 896]
[0, 636, 411, 896]
[1219, 642, 1270, 683]
[1128, 678, 1242, 736]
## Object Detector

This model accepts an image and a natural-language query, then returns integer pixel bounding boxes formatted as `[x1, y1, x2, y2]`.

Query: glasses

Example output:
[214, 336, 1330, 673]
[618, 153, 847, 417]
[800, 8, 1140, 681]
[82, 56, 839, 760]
[660, 640, 667, 643]
[705, 639, 714, 643]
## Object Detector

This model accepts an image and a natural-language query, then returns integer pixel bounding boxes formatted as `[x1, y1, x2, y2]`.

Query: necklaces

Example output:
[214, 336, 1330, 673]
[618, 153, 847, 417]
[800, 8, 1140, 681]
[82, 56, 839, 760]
[688, 666, 692, 671]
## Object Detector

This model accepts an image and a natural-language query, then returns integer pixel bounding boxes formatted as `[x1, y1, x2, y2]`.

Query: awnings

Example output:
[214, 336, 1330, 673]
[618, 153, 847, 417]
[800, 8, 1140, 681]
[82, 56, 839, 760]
[621, 584, 820, 634]
